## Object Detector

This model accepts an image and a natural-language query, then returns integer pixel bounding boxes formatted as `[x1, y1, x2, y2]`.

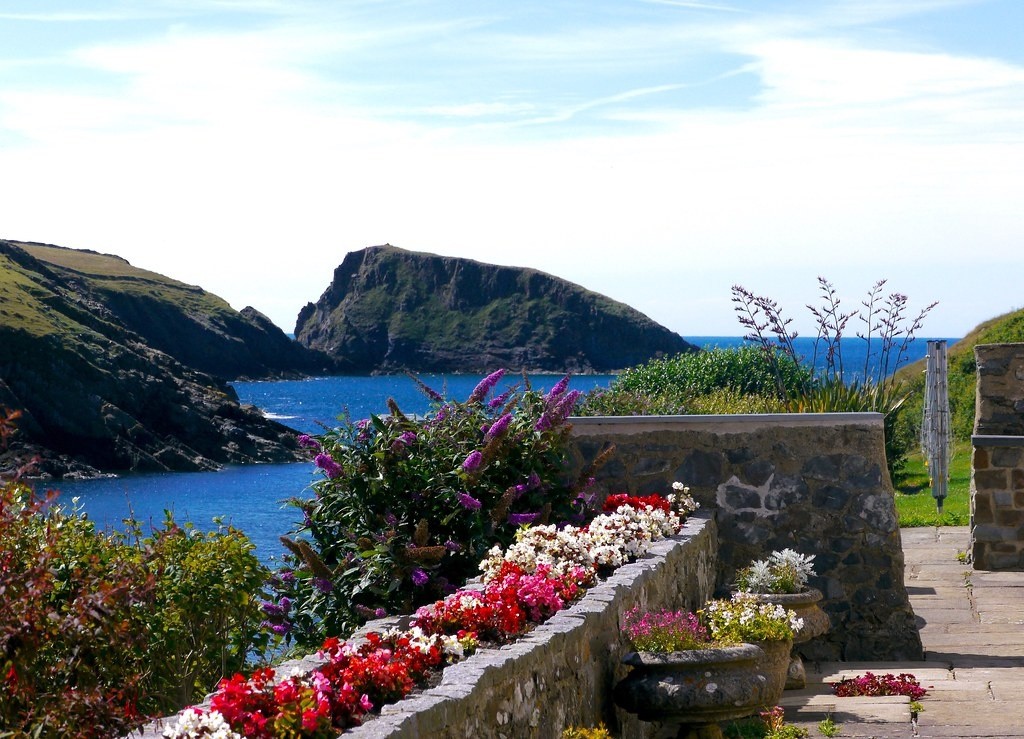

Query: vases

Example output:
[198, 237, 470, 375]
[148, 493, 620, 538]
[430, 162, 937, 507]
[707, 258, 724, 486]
[611, 639, 793, 724]
[733, 589, 832, 645]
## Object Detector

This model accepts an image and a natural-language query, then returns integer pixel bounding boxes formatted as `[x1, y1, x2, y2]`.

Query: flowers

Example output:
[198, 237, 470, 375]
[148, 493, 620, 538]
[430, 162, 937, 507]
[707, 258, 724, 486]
[618, 589, 805, 653]
[733, 547, 820, 594]
